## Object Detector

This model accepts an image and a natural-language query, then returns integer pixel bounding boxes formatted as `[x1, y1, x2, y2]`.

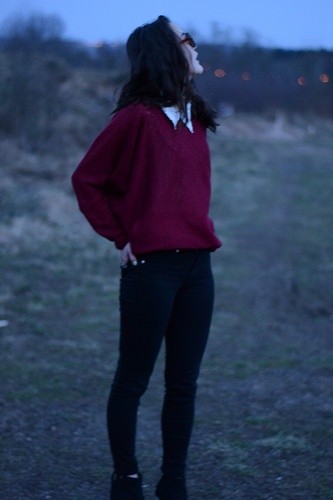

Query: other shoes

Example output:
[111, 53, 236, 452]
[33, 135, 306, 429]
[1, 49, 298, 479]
[155, 473, 189, 500]
[111, 470, 146, 499]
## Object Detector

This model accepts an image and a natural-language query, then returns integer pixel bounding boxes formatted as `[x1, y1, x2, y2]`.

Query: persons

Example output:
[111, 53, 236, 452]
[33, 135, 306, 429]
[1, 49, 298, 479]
[70, 15, 221, 500]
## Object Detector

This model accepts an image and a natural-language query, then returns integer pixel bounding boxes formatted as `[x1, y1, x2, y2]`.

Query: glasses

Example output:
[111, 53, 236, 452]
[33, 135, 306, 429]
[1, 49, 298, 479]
[179, 32, 196, 48]
